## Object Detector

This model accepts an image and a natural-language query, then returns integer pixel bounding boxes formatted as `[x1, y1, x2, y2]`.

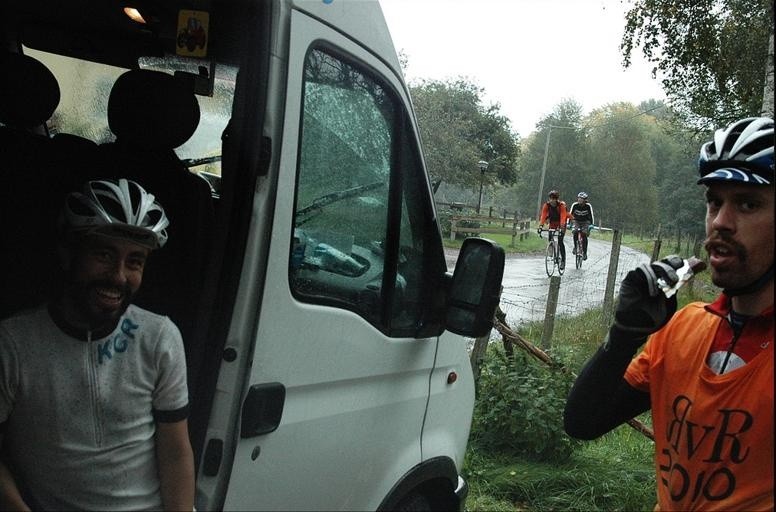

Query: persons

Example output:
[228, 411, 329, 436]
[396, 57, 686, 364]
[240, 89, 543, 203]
[561, 116, 775, 510]
[569, 191, 595, 260]
[0, 177, 199, 511]
[536, 187, 572, 271]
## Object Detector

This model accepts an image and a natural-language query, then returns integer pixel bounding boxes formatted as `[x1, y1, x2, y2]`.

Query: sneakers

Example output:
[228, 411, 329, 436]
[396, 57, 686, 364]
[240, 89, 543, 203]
[583, 254, 587, 260]
[560, 261, 566, 269]
[572, 248, 577, 255]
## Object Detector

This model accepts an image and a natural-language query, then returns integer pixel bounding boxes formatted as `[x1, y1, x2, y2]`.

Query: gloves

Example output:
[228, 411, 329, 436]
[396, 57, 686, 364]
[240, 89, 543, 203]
[566, 224, 571, 229]
[606, 253, 685, 350]
[537, 225, 543, 233]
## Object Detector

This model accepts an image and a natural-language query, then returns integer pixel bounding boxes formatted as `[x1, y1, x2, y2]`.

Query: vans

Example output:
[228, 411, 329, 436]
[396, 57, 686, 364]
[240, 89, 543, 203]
[0, 0, 505, 512]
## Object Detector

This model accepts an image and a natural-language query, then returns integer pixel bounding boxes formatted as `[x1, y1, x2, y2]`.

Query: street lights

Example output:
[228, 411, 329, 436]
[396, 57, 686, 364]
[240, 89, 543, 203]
[475, 157, 489, 210]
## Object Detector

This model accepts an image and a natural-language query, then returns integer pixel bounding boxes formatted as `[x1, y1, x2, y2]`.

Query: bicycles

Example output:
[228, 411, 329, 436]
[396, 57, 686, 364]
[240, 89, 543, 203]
[566, 222, 593, 270]
[537, 227, 567, 278]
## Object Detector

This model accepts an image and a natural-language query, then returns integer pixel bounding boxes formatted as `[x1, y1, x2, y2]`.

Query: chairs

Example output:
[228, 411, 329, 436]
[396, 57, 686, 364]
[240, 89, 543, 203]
[98, 69, 215, 235]
[0, 54, 61, 184]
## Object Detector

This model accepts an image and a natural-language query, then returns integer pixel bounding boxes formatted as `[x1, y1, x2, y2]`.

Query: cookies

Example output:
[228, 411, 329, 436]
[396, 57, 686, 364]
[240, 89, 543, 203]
[687, 255, 706, 274]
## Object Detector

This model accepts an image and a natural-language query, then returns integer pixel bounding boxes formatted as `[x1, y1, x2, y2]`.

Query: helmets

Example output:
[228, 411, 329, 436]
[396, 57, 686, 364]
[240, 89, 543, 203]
[54, 175, 171, 250]
[547, 189, 561, 198]
[696, 116, 776, 189]
[576, 191, 589, 199]
